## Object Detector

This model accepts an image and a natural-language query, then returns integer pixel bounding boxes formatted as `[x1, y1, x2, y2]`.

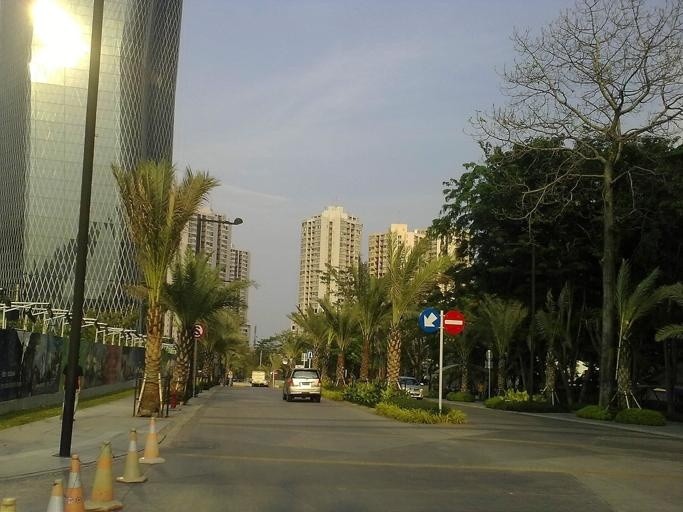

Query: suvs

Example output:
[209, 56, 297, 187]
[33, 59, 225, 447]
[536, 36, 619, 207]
[282, 367, 321, 402]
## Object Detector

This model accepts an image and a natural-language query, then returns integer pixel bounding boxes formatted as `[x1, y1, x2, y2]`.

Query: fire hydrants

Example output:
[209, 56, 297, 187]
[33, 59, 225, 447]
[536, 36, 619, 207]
[170, 390, 178, 409]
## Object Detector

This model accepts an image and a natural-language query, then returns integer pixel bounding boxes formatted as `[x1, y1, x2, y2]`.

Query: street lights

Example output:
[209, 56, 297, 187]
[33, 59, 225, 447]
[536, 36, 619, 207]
[184, 216, 244, 397]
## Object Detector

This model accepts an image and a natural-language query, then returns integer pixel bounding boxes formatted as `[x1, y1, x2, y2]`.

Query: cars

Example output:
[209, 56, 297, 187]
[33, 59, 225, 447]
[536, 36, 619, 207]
[396, 376, 423, 400]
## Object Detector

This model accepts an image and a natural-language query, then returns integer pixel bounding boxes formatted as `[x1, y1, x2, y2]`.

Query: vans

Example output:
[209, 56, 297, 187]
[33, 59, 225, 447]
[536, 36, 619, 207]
[251, 370, 267, 387]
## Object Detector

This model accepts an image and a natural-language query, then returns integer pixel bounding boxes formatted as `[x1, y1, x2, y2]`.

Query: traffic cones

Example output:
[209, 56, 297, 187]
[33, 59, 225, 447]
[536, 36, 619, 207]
[44, 479, 65, 512]
[138, 417, 165, 464]
[63, 454, 85, 511]
[0, 496, 16, 511]
[83, 440, 123, 511]
[115, 427, 147, 482]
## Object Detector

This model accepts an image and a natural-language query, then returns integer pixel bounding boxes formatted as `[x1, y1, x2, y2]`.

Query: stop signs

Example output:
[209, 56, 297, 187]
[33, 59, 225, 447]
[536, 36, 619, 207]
[442, 311, 464, 336]
